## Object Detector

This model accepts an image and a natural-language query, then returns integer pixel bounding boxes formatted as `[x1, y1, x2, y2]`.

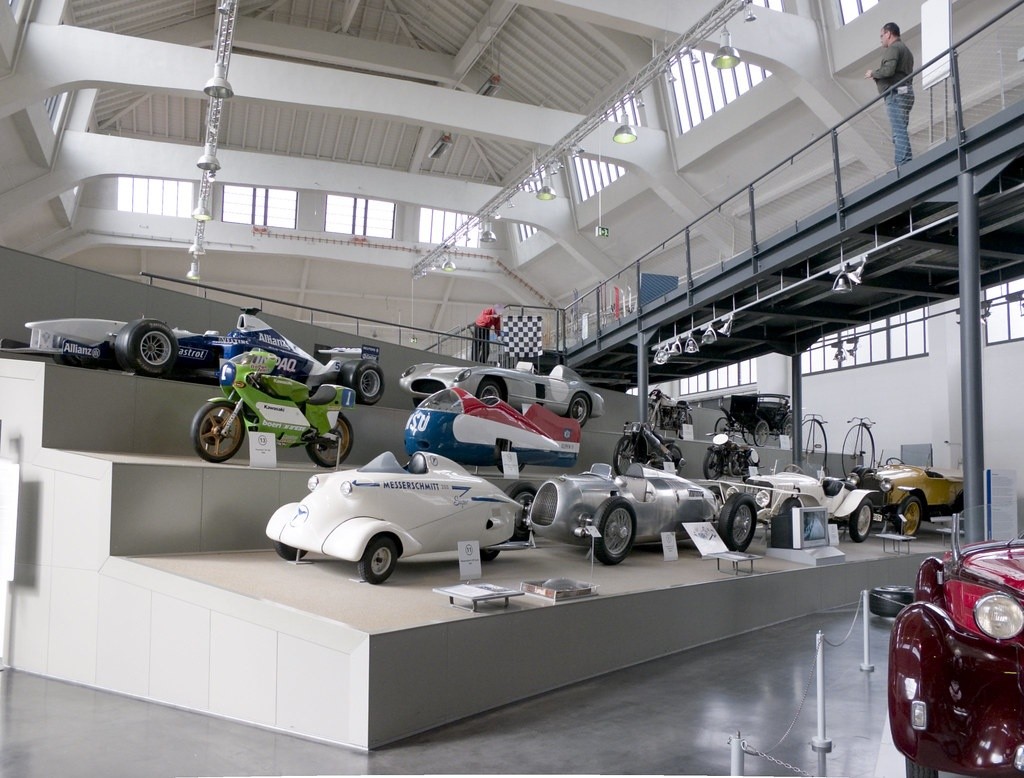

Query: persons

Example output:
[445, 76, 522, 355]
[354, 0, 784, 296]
[472, 303, 501, 363]
[865, 23, 913, 167]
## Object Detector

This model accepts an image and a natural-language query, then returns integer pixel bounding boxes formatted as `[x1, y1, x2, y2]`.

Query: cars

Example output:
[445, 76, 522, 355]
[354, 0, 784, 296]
[887, 504, 1024, 778]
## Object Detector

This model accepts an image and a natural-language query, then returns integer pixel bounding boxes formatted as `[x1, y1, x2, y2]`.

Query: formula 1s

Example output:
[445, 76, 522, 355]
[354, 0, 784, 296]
[1, 307, 386, 407]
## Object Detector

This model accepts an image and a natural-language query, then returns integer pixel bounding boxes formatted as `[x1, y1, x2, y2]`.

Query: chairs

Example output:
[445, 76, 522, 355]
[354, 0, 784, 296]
[822, 479, 844, 498]
[622, 476, 647, 501]
[408, 454, 426, 474]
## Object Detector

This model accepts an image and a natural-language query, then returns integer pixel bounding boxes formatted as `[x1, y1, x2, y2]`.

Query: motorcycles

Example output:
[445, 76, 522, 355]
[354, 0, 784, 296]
[702, 432, 765, 482]
[648, 389, 694, 440]
[192, 348, 356, 467]
[613, 421, 688, 478]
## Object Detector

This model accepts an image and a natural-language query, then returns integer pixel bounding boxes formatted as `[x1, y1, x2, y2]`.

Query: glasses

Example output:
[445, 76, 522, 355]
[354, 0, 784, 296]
[881, 31, 887, 37]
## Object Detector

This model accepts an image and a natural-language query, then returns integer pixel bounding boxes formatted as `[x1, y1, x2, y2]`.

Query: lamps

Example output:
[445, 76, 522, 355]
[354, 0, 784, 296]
[476, 25, 503, 96]
[422, 235, 471, 276]
[833, 339, 859, 361]
[537, 185, 557, 201]
[848, 258, 867, 282]
[956, 299, 1024, 325]
[831, 267, 854, 292]
[612, 97, 645, 143]
[427, 132, 453, 159]
[186, 262, 200, 279]
[191, 196, 212, 221]
[667, 7, 756, 83]
[549, 146, 585, 174]
[651, 315, 734, 364]
[197, 142, 222, 171]
[216, 4, 230, 14]
[188, 236, 206, 255]
[204, 62, 234, 99]
[481, 183, 533, 242]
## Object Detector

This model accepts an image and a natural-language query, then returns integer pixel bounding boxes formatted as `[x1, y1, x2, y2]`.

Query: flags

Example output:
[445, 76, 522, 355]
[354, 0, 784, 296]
[503, 316, 543, 357]
